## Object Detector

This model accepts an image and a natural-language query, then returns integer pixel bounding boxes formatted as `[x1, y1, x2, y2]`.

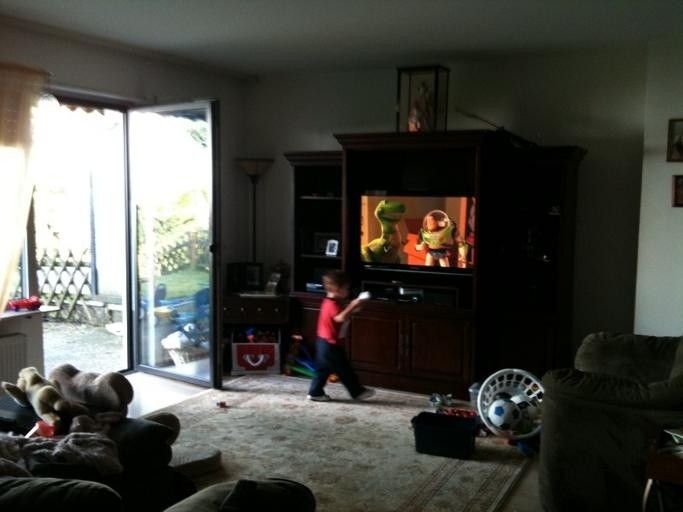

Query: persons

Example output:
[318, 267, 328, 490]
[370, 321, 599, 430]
[308, 270, 376, 401]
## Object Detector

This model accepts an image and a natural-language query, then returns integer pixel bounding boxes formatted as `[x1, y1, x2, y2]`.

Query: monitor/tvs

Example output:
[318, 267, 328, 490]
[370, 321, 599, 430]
[354, 189, 478, 278]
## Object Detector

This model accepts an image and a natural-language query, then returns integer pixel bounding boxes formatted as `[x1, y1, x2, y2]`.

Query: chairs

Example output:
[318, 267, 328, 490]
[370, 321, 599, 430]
[538, 334, 683, 512]
[139, 284, 210, 339]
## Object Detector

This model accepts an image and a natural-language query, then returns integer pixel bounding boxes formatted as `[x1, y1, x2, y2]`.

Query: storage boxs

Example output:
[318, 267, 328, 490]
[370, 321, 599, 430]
[230, 327, 282, 376]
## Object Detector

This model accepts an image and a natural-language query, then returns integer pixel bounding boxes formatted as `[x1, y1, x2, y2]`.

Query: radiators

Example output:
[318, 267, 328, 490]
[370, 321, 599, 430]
[0, 333, 29, 398]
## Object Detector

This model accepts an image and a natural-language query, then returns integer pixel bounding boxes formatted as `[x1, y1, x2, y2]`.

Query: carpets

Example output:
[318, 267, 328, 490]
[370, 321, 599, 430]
[138, 375, 531, 512]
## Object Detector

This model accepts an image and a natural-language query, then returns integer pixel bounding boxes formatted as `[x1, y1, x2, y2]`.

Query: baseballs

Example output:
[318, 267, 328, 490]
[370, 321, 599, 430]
[519, 418, 533, 434]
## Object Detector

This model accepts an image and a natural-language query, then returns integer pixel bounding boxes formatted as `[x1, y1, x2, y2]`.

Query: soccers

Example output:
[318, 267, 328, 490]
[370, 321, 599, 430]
[510, 395, 539, 422]
[488, 398, 522, 433]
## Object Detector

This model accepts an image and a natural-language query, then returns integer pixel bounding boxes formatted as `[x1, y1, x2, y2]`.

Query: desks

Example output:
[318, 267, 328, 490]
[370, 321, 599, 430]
[222, 288, 291, 369]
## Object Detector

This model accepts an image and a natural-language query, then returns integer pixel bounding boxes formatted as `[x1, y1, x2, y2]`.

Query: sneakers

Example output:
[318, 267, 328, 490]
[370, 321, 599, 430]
[306, 395, 330, 401]
[355, 388, 375, 399]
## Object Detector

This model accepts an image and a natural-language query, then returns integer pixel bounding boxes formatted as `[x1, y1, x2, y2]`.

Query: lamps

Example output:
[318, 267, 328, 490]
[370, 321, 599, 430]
[235, 158, 274, 263]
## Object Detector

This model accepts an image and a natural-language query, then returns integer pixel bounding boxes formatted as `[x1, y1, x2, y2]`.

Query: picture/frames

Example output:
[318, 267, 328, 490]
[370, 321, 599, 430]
[667, 119, 683, 162]
[672, 175, 683, 207]
[243, 263, 263, 291]
